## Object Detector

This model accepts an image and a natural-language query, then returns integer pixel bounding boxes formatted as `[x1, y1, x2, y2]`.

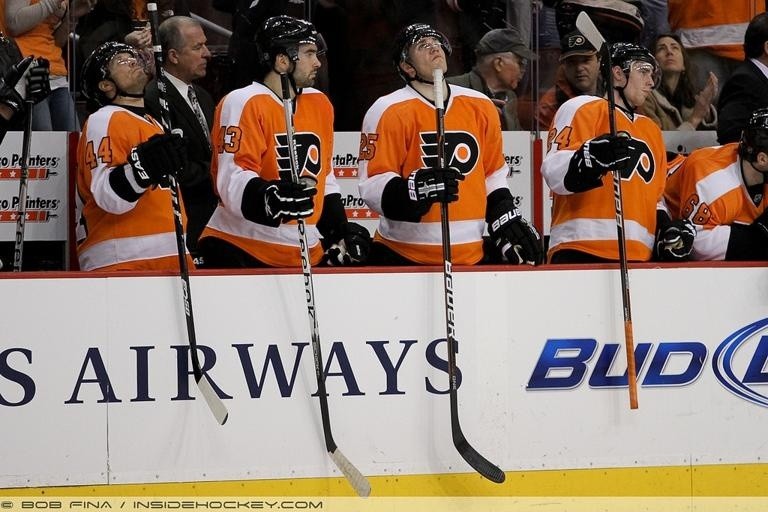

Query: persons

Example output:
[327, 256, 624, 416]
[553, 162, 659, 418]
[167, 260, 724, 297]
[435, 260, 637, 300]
[0, 0, 768, 273]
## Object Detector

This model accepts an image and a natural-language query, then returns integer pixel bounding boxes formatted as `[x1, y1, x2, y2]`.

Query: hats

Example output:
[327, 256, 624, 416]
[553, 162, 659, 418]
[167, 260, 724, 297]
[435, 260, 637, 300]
[558, 30, 598, 62]
[476, 29, 541, 60]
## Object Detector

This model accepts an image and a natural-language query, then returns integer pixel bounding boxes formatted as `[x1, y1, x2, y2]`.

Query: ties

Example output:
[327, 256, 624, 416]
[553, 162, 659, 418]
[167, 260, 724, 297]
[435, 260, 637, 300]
[188, 86, 212, 153]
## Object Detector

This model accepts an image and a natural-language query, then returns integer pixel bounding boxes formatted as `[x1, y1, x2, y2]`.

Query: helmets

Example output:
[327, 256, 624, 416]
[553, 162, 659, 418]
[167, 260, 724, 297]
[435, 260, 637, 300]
[601, 41, 663, 95]
[391, 23, 452, 86]
[253, 16, 329, 68]
[79, 41, 147, 108]
[740, 108, 768, 163]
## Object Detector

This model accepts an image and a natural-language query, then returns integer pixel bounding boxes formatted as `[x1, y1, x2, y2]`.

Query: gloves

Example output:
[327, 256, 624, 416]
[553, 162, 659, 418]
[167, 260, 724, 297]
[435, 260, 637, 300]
[0, 55, 51, 114]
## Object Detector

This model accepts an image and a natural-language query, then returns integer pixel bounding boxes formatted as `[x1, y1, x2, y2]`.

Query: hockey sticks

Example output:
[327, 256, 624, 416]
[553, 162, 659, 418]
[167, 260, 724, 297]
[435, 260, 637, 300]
[433, 69, 505, 484]
[575, 11, 637, 408]
[279, 72, 371, 497]
[147, 3, 228, 425]
